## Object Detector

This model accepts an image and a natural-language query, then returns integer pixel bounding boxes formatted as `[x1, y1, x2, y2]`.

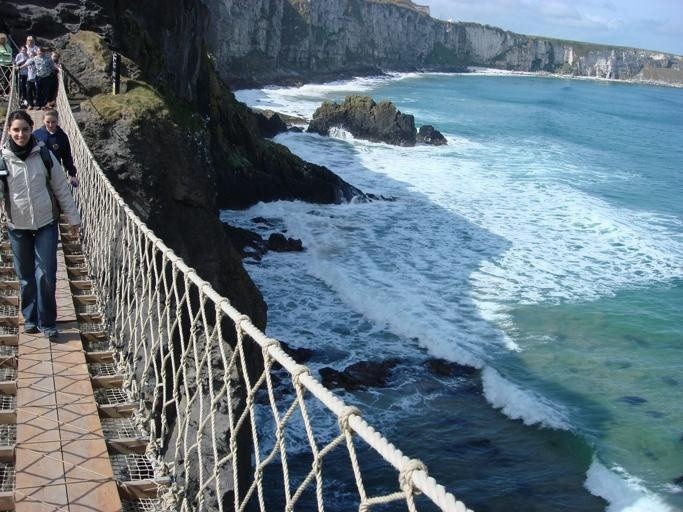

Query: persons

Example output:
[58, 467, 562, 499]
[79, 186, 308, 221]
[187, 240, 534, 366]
[0, 110, 81, 338]
[0, 33, 60, 111]
[32, 109, 79, 187]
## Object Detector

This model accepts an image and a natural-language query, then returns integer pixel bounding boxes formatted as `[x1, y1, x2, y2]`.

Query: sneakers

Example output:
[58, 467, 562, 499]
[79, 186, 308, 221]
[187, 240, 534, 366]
[25, 322, 38, 333]
[43, 326, 58, 338]
